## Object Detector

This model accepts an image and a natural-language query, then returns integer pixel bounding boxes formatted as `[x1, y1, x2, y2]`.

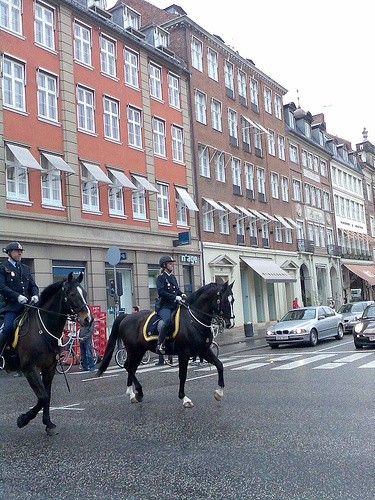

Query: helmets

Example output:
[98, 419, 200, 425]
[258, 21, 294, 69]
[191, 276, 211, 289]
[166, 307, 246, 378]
[5, 242, 26, 252]
[160, 255, 176, 266]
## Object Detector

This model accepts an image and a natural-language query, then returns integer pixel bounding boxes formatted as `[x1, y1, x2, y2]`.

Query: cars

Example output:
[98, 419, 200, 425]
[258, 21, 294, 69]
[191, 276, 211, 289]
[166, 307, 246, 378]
[263, 306, 346, 348]
[337, 301, 375, 350]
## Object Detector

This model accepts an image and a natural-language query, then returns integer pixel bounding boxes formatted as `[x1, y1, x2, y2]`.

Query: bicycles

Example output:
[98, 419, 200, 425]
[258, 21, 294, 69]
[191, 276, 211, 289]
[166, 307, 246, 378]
[115, 346, 152, 368]
[55, 336, 99, 374]
[162, 339, 221, 367]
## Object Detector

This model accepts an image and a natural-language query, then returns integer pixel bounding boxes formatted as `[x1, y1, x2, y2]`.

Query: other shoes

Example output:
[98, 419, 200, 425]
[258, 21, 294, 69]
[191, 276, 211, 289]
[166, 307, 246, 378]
[155, 363, 164, 365]
[89, 367, 95, 371]
[80, 368, 88, 371]
[157, 345, 165, 354]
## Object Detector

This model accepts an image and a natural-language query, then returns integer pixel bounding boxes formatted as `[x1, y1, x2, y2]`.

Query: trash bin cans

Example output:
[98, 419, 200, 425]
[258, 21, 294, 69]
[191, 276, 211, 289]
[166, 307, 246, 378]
[244, 323, 254, 337]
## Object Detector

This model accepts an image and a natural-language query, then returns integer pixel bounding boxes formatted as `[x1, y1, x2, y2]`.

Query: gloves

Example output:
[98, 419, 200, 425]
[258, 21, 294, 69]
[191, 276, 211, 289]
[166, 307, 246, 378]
[176, 296, 182, 300]
[182, 294, 187, 298]
[31, 295, 39, 303]
[18, 295, 28, 304]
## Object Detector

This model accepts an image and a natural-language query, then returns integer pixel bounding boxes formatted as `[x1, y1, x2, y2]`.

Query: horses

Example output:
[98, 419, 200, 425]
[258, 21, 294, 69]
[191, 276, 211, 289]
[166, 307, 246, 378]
[0, 271, 93, 437]
[94, 279, 235, 409]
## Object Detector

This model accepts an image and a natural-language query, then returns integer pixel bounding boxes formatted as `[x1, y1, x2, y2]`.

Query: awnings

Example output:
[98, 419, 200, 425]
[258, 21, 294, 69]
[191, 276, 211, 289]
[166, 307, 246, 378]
[239, 256, 297, 283]
[343, 263, 375, 286]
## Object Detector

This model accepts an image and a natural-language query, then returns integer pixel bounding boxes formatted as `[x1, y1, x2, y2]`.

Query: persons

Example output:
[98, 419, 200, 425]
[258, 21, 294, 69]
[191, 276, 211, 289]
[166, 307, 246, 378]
[132, 306, 140, 313]
[155, 255, 188, 355]
[292, 297, 300, 309]
[78, 314, 95, 372]
[0, 241, 39, 354]
